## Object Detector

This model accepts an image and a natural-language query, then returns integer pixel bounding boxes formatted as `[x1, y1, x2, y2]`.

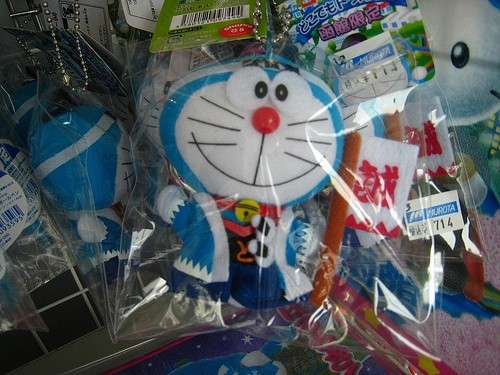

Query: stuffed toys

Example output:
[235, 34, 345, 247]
[1, 29, 387, 319]
